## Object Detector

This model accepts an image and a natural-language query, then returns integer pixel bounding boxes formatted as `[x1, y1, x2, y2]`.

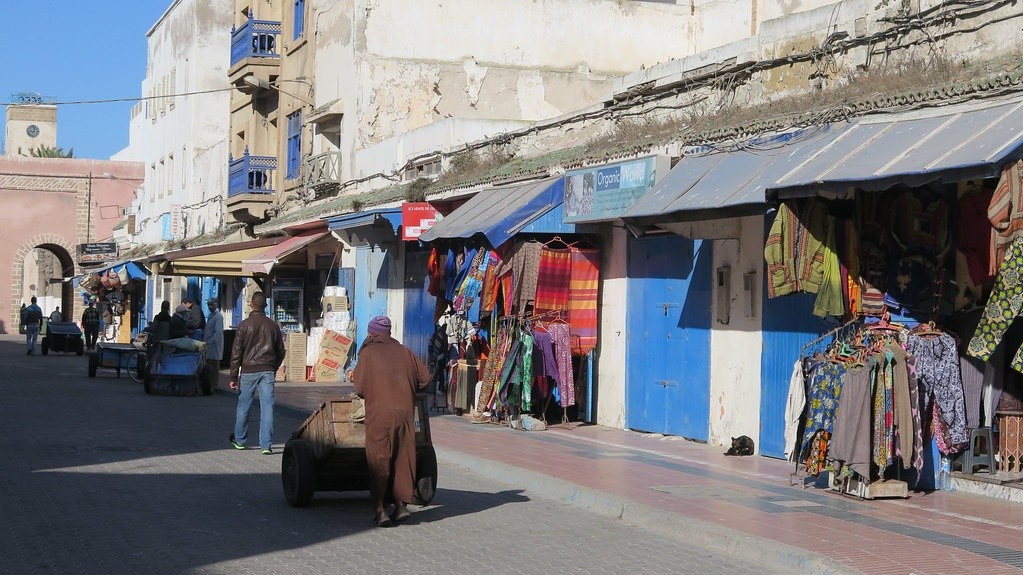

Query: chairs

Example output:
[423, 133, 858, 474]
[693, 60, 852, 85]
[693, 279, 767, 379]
[248, 170, 267, 195]
[252, 34, 275, 59]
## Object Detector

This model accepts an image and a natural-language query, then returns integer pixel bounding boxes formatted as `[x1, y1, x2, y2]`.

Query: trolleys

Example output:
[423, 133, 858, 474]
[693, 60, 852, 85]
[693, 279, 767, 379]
[282, 391, 438, 509]
[41, 320, 84, 357]
[87, 342, 144, 378]
[141, 339, 217, 394]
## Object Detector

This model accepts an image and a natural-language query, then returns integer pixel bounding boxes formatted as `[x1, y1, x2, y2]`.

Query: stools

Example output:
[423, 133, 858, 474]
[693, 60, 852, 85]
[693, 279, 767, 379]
[993, 409, 1023, 472]
[950, 426, 995, 475]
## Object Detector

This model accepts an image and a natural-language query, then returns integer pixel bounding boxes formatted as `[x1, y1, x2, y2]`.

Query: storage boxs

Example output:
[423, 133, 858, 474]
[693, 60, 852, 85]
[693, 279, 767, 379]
[283, 295, 353, 383]
[828, 471, 907, 499]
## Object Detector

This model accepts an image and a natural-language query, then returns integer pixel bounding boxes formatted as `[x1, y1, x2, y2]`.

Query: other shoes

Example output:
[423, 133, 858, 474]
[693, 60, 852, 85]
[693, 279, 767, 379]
[391, 507, 411, 522]
[377, 512, 392, 527]
[27, 350, 31, 355]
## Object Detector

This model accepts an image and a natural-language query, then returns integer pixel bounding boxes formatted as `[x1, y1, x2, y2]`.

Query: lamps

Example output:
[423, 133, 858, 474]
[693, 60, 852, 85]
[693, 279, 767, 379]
[244, 76, 314, 107]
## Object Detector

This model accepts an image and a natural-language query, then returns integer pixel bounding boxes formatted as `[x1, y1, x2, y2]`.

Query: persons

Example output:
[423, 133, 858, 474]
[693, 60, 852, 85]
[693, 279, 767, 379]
[230, 292, 286, 454]
[20, 303, 27, 333]
[23, 296, 43, 355]
[169, 305, 191, 338]
[181, 298, 206, 341]
[354, 316, 430, 527]
[201, 298, 225, 394]
[145, 301, 171, 337]
[50, 306, 61, 322]
[82, 301, 100, 350]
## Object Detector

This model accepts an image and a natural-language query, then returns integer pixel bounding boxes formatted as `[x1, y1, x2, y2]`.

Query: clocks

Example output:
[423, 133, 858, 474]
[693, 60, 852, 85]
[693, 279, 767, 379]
[26, 125, 40, 138]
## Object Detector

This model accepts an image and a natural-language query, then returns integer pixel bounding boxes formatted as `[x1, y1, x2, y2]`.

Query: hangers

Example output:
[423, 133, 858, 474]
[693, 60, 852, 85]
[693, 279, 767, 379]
[524, 235, 604, 250]
[918, 321, 943, 337]
[498, 310, 571, 335]
[799, 313, 909, 370]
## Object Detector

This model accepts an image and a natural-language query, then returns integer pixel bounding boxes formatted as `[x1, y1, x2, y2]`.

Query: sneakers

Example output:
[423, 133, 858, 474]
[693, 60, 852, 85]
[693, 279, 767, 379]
[262, 449, 273, 455]
[229, 432, 246, 450]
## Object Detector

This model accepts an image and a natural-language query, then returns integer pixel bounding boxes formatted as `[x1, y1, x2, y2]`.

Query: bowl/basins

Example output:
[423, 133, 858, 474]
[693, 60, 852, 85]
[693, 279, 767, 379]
[133, 342, 152, 349]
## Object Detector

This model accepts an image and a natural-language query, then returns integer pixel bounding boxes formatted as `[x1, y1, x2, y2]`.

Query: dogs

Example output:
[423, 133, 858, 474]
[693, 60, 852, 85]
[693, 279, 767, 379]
[723, 436, 754, 456]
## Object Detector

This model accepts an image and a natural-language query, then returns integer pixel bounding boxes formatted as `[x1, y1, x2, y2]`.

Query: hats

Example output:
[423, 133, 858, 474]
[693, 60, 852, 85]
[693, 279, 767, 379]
[368, 315, 391, 335]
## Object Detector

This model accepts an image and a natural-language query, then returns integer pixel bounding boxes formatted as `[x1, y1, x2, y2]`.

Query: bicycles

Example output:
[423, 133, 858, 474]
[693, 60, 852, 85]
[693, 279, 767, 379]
[128, 338, 152, 383]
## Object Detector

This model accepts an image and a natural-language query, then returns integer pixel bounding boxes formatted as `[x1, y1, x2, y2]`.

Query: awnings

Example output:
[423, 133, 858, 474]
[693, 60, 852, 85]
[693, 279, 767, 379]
[621, 101, 1023, 226]
[417, 176, 564, 249]
[242, 229, 331, 275]
